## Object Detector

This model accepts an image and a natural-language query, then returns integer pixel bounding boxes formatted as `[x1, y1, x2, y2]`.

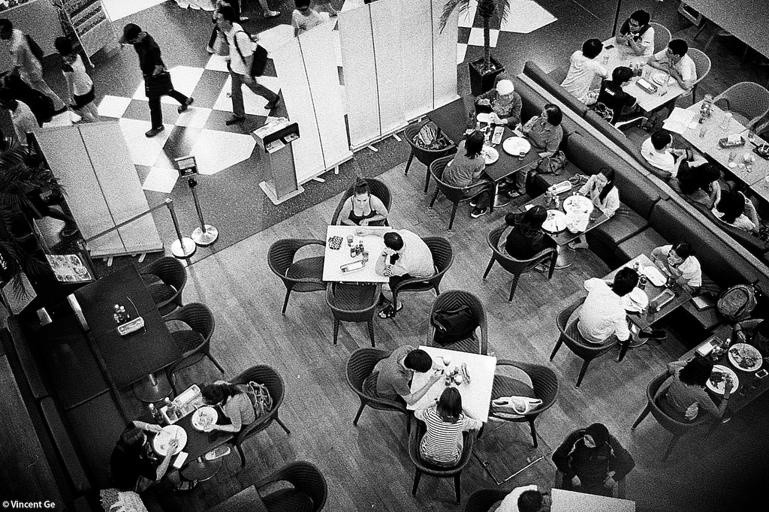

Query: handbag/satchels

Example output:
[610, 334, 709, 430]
[538, 150, 568, 178]
[413, 120, 448, 151]
[491, 395, 543, 416]
[431, 304, 477, 346]
[23, 33, 45, 60]
[143, 72, 174, 99]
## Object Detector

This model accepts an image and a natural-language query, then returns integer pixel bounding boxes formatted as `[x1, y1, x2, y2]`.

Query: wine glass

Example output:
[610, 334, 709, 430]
[430, 356, 464, 392]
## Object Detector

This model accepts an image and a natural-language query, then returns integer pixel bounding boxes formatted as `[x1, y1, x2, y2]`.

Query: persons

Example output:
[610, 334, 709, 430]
[356, 227, 435, 319]
[442, 128, 490, 217]
[0, 17, 100, 235]
[552, 423, 635, 497]
[341, 179, 389, 226]
[560, 7, 769, 421]
[206, 1, 337, 53]
[474, 80, 521, 126]
[508, 103, 564, 199]
[493, 485, 551, 512]
[363, 345, 447, 406]
[504, 205, 557, 272]
[119, 23, 194, 137]
[217, 7, 280, 125]
[194, 381, 256, 460]
[414, 388, 483, 471]
[113, 421, 197, 499]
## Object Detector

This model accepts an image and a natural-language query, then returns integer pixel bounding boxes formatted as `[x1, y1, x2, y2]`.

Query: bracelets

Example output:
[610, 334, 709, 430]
[541, 493, 548, 496]
[145, 424, 149, 430]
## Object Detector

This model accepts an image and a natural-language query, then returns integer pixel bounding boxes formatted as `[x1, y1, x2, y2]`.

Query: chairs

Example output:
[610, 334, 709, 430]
[41, 391, 132, 495]
[227, 363, 290, 468]
[137, 256, 187, 317]
[163, 303, 225, 394]
[7, 292, 111, 412]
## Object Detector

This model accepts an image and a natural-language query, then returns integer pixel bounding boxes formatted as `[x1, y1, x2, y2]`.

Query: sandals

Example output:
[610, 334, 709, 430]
[174, 478, 199, 493]
[499, 179, 515, 189]
[378, 301, 403, 319]
[508, 185, 527, 199]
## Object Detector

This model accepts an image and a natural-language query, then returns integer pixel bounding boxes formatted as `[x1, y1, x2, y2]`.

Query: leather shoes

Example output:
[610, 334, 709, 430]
[568, 241, 590, 250]
[205, 445, 230, 460]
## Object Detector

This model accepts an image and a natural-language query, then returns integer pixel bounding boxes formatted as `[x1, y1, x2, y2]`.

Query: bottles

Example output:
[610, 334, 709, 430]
[164, 396, 176, 413]
[111, 302, 128, 324]
[148, 403, 159, 419]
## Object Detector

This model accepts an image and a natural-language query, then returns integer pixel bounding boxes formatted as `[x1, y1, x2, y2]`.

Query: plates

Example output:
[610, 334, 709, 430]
[477, 111, 532, 165]
[192, 407, 218, 431]
[152, 425, 188, 456]
[540, 180, 595, 233]
[704, 340, 764, 396]
[620, 259, 677, 313]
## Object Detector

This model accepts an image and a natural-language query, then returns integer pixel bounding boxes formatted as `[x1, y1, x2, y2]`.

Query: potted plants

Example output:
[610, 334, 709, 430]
[438, 0, 510, 97]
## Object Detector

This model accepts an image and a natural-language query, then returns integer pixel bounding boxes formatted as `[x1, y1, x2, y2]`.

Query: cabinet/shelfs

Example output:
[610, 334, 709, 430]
[49, 0, 125, 68]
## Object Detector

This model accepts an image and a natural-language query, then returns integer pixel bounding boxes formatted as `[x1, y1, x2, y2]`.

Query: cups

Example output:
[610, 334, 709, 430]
[603, 44, 675, 96]
[696, 92, 769, 192]
[346, 225, 370, 263]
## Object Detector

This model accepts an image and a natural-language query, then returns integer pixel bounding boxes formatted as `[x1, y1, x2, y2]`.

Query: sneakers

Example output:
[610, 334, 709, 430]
[264, 95, 280, 109]
[470, 198, 487, 219]
[177, 98, 193, 113]
[226, 115, 246, 125]
[145, 126, 164, 138]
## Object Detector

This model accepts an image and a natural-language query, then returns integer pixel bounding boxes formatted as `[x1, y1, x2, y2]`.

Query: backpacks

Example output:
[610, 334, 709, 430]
[715, 278, 761, 324]
[234, 30, 269, 79]
